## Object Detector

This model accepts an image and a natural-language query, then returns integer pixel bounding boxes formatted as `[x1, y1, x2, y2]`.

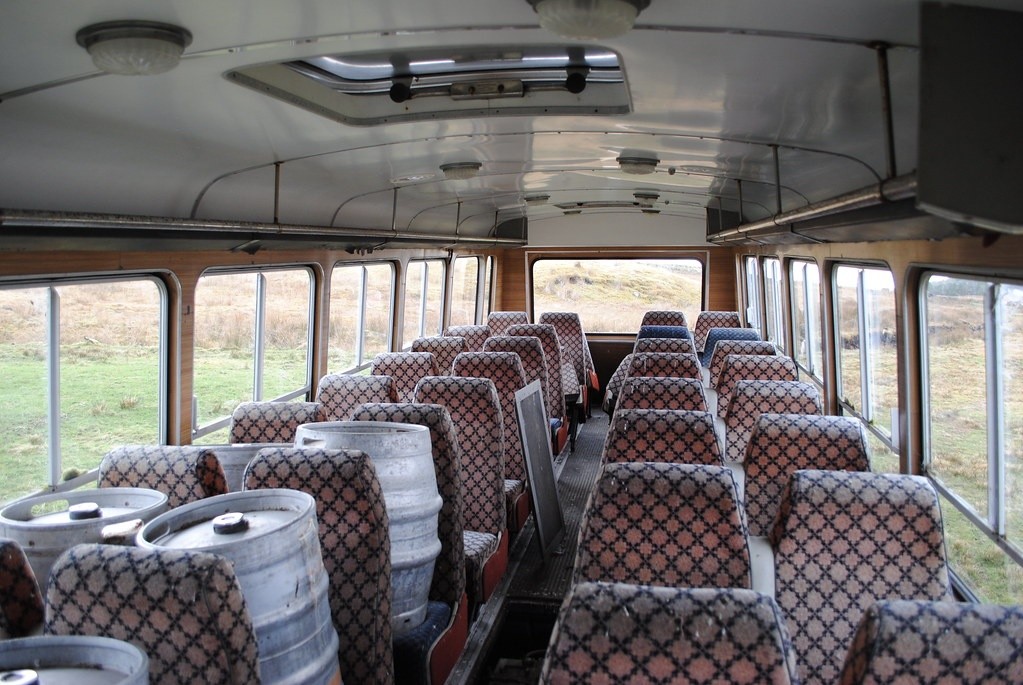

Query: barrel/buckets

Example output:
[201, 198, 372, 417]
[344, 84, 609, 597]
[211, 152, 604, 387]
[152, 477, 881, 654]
[0, 486, 168, 607]
[292, 420, 444, 635]
[134, 488, 345, 685]
[0, 635, 151, 685]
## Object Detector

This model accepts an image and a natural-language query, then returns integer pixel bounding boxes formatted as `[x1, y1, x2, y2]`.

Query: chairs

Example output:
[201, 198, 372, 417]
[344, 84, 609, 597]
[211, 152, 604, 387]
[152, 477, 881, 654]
[0, 311, 1023, 685]
